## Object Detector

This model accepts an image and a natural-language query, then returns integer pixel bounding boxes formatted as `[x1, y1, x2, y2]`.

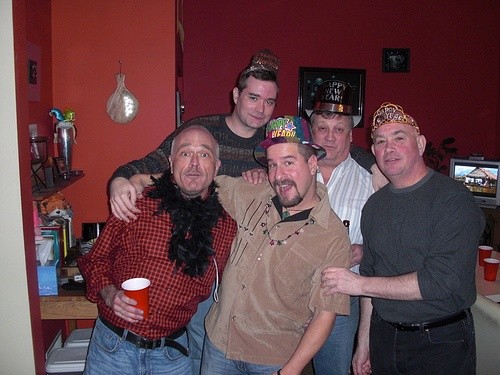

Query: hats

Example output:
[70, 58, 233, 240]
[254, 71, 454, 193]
[313, 80, 356, 115]
[254, 115, 327, 167]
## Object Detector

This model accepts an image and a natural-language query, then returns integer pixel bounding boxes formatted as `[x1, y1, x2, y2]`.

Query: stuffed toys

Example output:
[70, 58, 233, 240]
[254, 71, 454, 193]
[40, 191, 67, 220]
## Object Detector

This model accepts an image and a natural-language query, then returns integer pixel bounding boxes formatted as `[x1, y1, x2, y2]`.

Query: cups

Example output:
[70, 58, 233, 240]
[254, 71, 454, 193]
[478, 246, 493, 266]
[121, 278, 151, 319]
[483, 258, 500, 282]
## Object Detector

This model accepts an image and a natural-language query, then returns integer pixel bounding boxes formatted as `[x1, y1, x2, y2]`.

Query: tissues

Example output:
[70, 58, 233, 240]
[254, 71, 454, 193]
[36, 239, 60, 296]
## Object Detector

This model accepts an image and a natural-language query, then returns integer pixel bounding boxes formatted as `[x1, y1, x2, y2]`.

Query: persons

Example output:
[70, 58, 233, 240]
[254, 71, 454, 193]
[76, 125, 237, 375]
[130, 115, 353, 375]
[108, 51, 391, 223]
[241, 82, 375, 375]
[321, 102, 486, 375]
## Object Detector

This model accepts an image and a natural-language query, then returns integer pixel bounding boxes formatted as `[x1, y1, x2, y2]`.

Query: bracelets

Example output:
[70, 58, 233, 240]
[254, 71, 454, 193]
[277, 369, 281, 375]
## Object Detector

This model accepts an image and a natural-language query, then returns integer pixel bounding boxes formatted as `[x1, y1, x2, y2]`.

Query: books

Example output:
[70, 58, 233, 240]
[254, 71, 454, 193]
[37, 217, 73, 273]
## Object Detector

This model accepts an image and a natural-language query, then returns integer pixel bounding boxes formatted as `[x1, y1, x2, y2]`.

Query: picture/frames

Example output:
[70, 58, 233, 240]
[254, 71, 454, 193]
[297, 66, 367, 129]
[382, 47, 410, 73]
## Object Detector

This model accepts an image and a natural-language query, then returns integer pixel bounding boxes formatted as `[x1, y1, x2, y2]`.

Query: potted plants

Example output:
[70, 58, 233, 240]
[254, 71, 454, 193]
[422, 136, 458, 173]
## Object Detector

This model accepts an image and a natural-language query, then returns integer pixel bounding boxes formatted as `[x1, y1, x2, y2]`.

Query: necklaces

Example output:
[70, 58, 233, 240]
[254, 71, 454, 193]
[282, 207, 303, 220]
[261, 201, 317, 247]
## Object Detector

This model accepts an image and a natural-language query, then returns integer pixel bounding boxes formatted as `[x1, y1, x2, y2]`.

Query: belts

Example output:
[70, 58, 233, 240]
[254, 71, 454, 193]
[99, 316, 190, 357]
[375, 307, 472, 333]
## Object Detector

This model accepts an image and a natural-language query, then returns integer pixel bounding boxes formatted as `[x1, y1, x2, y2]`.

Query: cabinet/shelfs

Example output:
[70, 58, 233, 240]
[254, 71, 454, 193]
[30, 169, 99, 321]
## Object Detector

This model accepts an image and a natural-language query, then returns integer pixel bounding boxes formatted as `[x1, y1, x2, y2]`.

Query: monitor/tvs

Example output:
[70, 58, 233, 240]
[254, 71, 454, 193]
[449, 159, 500, 210]
